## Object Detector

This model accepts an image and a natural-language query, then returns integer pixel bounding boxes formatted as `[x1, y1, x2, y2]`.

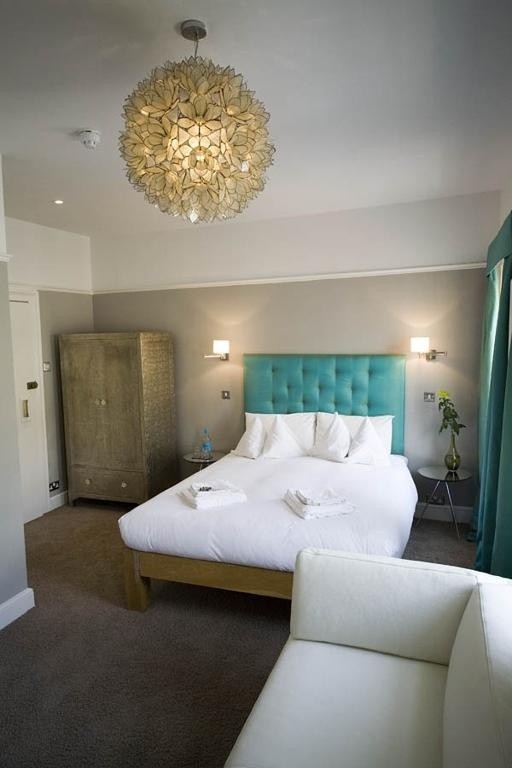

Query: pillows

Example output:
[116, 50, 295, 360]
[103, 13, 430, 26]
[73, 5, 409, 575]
[345, 413, 388, 464]
[261, 413, 306, 459]
[309, 413, 351, 463]
[311, 411, 395, 462]
[244, 411, 317, 455]
[229, 418, 268, 460]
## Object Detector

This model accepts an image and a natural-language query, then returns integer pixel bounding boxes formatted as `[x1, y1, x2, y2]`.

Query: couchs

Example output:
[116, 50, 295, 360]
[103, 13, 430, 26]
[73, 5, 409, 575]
[215, 546, 512, 767]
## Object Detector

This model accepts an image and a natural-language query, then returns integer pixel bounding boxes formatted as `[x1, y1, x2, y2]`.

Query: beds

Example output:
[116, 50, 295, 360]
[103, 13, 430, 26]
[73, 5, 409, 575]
[115, 351, 418, 614]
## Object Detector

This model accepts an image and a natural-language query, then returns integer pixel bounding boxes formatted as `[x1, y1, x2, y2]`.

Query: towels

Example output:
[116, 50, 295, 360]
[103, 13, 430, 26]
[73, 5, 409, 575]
[279, 477, 356, 520]
[181, 478, 246, 510]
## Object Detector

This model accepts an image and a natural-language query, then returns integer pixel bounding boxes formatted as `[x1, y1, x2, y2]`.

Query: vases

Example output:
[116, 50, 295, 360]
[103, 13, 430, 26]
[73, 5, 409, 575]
[442, 434, 461, 471]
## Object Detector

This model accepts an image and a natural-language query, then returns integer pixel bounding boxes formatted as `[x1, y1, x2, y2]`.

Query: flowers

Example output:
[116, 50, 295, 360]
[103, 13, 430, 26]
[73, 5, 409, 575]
[435, 388, 470, 469]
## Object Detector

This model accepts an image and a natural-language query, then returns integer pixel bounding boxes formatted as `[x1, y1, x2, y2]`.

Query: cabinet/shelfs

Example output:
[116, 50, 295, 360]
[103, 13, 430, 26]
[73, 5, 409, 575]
[54, 330, 182, 511]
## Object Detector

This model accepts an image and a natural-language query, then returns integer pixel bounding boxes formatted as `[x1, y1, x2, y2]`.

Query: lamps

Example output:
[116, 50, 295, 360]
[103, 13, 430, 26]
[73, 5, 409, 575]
[203, 339, 232, 362]
[120, 19, 276, 228]
[411, 335, 450, 363]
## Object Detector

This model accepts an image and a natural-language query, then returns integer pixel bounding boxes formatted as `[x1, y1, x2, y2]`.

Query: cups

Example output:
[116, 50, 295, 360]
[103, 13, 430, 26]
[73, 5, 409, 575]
[192, 442, 200, 458]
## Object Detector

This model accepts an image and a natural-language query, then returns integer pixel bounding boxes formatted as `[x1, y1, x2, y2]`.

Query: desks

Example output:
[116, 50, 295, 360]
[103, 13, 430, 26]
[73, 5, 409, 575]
[415, 465, 474, 542]
[183, 451, 228, 472]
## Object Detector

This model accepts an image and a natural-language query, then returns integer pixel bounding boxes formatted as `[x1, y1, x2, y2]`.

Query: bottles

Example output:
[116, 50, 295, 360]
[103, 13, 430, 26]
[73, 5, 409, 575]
[200, 427, 213, 459]
